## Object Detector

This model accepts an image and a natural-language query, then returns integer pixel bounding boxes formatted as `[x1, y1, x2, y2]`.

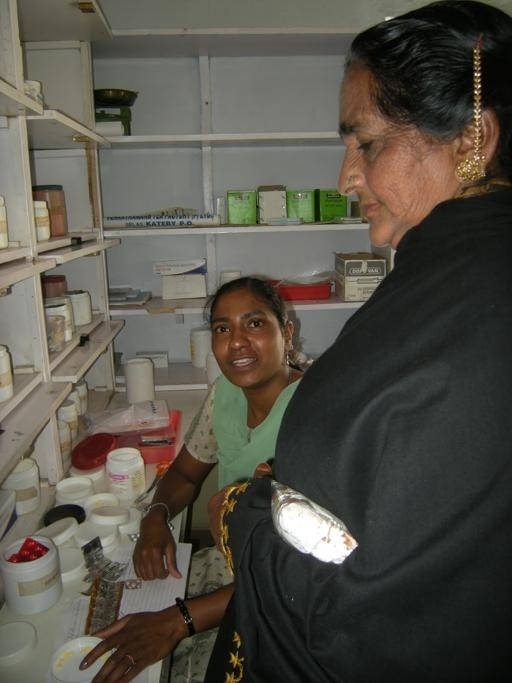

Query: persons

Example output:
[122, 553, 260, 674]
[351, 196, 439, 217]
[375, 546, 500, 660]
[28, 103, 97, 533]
[206, 1, 510, 683]
[77, 271, 309, 682]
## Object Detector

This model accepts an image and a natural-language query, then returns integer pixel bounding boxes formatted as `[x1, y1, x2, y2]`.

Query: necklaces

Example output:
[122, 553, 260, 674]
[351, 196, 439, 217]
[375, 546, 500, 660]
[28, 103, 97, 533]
[248, 368, 294, 421]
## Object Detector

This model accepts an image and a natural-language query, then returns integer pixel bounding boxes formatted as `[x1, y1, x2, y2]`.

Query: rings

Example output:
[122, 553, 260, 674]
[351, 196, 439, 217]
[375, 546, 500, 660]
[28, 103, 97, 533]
[124, 653, 135, 665]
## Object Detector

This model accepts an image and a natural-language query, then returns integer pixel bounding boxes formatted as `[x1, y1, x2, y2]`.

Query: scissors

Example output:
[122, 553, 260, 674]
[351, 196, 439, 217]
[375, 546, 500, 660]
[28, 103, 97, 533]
[147, 462, 171, 496]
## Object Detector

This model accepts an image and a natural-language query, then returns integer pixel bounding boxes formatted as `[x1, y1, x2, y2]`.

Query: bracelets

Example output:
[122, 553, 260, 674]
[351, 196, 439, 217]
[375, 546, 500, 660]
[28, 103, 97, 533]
[144, 501, 174, 532]
[174, 597, 196, 637]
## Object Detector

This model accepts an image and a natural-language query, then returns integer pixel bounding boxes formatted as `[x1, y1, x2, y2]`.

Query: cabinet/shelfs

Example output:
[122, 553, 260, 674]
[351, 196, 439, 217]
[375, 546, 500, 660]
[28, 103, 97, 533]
[2, 0, 114, 616]
[94, 30, 398, 393]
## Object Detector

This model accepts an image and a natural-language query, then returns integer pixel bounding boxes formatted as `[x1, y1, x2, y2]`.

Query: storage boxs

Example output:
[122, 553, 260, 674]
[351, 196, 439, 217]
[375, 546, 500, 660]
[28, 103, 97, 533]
[282, 188, 315, 226]
[223, 188, 259, 225]
[332, 250, 388, 303]
[312, 189, 347, 225]
[254, 182, 288, 227]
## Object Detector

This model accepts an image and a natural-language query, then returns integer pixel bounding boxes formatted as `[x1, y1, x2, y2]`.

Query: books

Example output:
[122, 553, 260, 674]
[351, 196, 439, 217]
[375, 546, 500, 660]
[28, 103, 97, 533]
[110, 286, 153, 306]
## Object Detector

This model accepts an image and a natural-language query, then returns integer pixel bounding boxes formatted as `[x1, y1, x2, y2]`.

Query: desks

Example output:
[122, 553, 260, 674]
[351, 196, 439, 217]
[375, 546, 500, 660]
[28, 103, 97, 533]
[0, 390, 210, 683]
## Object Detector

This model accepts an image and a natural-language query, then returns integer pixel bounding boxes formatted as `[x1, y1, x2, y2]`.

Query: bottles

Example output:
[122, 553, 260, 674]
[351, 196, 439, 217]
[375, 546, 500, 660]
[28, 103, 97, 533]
[73, 381, 88, 417]
[42, 274, 93, 353]
[1, 458, 41, 516]
[105, 446, 146, 502]
[124, 357, 156, 405]
[2, 535, 63, 616]
[0, 345, 14, 403]
[57, 420, 73, 474]
[57, 400, 78, 441]
[33, 184, 68, 241]
[0, 196, 9, 250]
[190, 326, 213, 368]
